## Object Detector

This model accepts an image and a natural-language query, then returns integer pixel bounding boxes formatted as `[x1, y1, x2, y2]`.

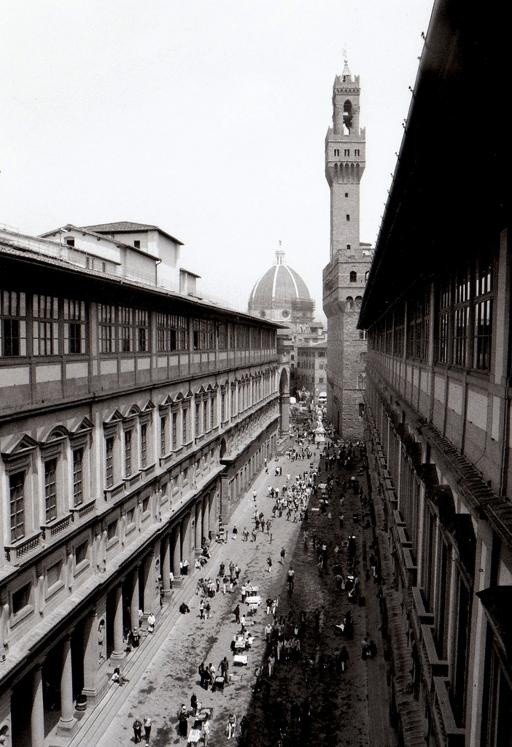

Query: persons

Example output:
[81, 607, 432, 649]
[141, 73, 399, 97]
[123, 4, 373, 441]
[258, 596, 378, 698]
[231, 467, 320, 544]
[112, 610, 156, 685]
[165, 541, 257, 654]
[266, 526, 379, 603]
[131, 657, 239, 747]
[44, 680, 58, 710]
[263, 423, 317, 475]
[318, 440, 370, 531]
